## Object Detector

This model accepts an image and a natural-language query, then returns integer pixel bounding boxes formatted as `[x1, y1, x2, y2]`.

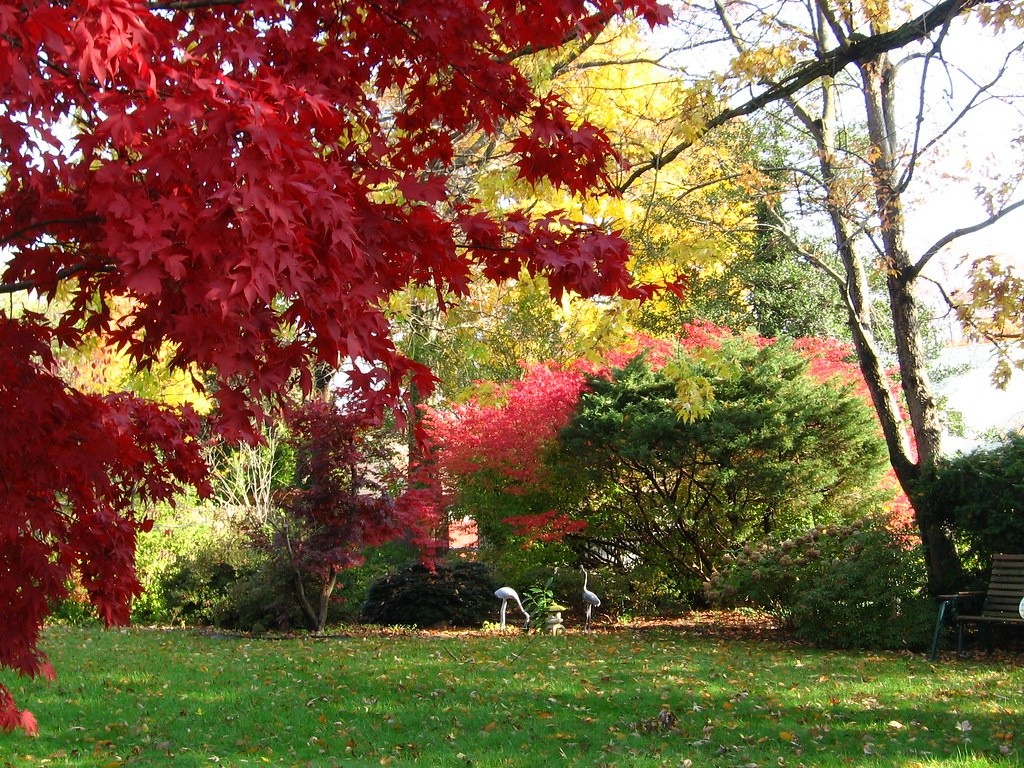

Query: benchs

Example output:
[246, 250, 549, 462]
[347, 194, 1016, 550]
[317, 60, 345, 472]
[930, 553, 1024, 660]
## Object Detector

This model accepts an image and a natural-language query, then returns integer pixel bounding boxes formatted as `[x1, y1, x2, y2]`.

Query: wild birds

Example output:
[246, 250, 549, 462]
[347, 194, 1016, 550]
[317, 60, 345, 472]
[494, 587, 530, 626]
[579, 564, 601, 633]
